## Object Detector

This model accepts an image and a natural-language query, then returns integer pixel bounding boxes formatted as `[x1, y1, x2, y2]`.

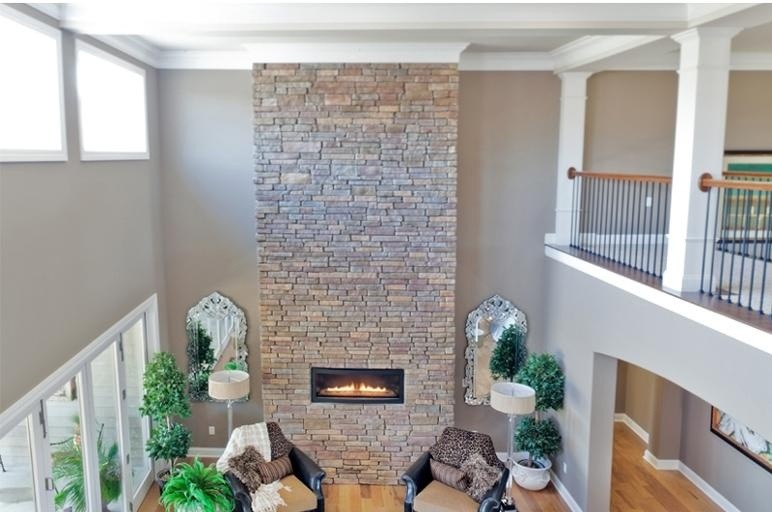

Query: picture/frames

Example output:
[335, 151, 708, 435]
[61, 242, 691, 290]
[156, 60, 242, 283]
[715, 147, 772, 243]
[709, 403, 772, 473]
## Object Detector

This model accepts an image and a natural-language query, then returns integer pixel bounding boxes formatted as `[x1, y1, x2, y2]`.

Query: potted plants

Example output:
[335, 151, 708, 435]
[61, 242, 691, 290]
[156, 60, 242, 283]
[510, 350, 562, 492]
[139, 347, 192, 496]
[157, 456, 237, 511]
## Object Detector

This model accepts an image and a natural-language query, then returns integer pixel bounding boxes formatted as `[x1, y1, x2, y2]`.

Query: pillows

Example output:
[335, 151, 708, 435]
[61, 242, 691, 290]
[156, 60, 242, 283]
[457, 452, 502, 502]
[428, 458, 469, 491]
[227, 446, 265, 493]
[257, 452, 294, 484]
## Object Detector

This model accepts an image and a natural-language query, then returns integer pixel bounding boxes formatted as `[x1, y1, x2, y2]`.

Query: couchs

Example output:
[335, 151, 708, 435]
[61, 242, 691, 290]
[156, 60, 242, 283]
[216, 420, 329, 512]
[400, 424, 510, 511]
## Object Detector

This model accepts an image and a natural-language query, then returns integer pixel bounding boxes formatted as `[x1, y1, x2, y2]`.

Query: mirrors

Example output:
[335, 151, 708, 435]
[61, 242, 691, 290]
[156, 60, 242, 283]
[460, 292, 529, 405]
[185, 289, 250, 403]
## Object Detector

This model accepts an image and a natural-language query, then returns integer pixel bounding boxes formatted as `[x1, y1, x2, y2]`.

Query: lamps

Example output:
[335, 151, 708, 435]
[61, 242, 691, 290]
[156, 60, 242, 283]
[208, 369, 251, 442]
[488, 381, 536, 511]
